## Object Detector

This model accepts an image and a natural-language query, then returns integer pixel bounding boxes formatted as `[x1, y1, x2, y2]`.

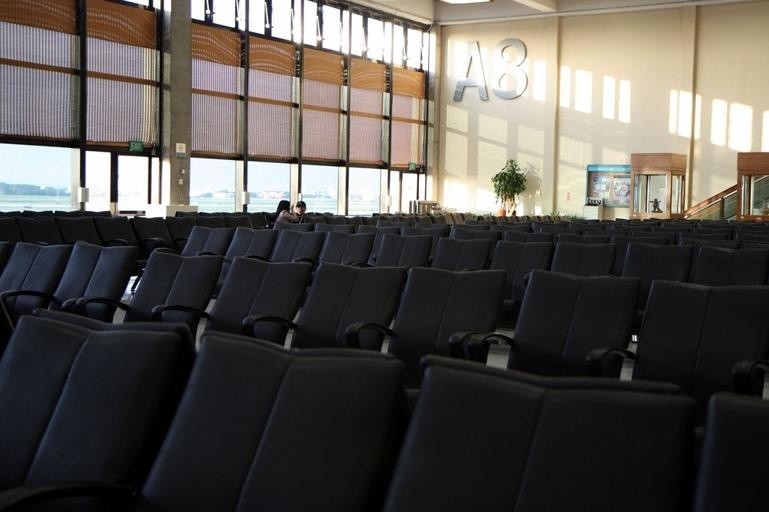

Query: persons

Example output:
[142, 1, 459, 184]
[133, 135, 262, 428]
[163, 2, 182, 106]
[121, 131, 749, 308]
[277, 200, 290, 212]
[296, 201, 306, 216]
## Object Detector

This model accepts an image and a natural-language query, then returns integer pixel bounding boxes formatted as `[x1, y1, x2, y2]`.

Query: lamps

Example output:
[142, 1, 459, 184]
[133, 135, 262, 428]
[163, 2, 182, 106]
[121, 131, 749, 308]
[76, 187, 89, 211]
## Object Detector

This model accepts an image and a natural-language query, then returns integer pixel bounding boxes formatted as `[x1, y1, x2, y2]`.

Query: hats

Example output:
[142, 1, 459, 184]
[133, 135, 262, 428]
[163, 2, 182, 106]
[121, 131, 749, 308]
[296, 201, 306, 207]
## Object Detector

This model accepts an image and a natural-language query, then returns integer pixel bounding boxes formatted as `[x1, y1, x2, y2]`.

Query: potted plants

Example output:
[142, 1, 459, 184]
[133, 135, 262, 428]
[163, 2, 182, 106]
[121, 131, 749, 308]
[492, 158, 526, 217]
